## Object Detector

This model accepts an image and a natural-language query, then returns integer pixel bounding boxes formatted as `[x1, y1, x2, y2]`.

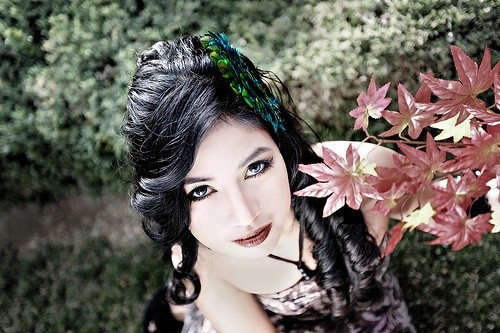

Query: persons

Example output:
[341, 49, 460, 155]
[120, 31, 467, 333]
